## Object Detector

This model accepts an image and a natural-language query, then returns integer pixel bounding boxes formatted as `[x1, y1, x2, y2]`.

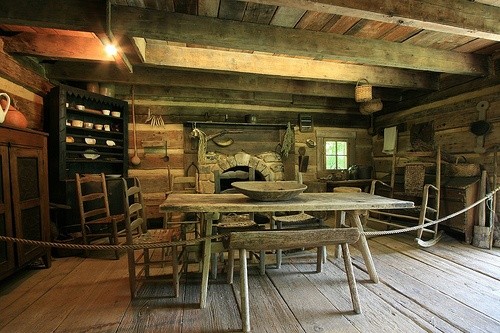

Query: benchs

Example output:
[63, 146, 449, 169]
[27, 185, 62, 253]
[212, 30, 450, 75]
[222, 227, 362, 333]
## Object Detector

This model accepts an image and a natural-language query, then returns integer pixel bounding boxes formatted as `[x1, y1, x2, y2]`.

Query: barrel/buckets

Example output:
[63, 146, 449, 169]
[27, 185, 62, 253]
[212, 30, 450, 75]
[99, 82, 114, 98]
[87, 82, 99, 93]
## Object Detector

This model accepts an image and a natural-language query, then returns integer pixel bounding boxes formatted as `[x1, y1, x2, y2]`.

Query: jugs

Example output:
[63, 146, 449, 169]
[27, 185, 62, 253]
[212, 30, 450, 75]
[0, 93, 10, 123]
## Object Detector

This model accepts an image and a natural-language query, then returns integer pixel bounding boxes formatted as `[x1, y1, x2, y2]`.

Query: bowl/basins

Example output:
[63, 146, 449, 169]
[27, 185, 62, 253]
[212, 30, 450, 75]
[84, 123, 94, 129]
[102, 110, 110, 115]
[84, 153, 100, 159]
[95, 125, 103, 130]
[66, 137, 74, 142]
[71, 120, 83, 127]
[230, 181, 308, 202]
[76, 105, 84, 110]
[85, 138, 96, 144]
[106, 141, 114, 146]
[244, 115, 257, 123]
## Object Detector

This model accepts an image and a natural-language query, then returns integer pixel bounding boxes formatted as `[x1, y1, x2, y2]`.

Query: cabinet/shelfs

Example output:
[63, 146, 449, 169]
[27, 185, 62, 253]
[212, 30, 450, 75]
[0, 122, 51, 280]
[47, 84, 128, 238]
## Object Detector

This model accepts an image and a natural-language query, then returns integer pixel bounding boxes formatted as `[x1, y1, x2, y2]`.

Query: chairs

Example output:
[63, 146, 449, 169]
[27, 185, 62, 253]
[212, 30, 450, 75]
[212, 166, 266, 281]
[363, 146, 447, 247]
[75, 171, 139, 260]
[122, 177, 188, 301]
[267, 172, 326, 269]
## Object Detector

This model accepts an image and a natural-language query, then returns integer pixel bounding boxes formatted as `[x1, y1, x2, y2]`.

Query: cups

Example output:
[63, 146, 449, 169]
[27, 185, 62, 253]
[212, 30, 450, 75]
[112, 112, 120, 117]
[104, 125, 110, 131]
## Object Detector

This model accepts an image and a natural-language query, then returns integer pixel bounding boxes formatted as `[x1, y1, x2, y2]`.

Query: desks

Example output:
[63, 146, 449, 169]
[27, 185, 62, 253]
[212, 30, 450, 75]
[158, 193, 415, 308]
